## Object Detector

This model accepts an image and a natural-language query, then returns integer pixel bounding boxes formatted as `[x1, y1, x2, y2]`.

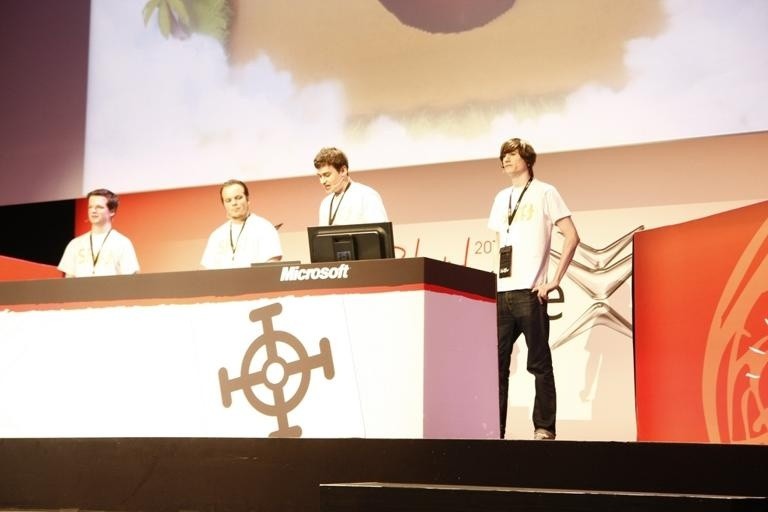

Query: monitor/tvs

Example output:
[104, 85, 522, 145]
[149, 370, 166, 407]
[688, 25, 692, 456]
[308, 222, 395, 263]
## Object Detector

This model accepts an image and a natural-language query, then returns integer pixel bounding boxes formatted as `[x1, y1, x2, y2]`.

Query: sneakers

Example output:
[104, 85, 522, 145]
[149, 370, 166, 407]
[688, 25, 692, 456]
[534, 428, 556, 440]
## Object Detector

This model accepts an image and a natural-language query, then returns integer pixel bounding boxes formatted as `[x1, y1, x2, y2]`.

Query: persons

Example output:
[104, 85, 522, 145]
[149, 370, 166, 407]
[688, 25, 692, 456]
[314, 146, 390, 227]
[486, 138, 580, 440]
[201, 179, 283, 269]
[56, 189, 141, 278]
